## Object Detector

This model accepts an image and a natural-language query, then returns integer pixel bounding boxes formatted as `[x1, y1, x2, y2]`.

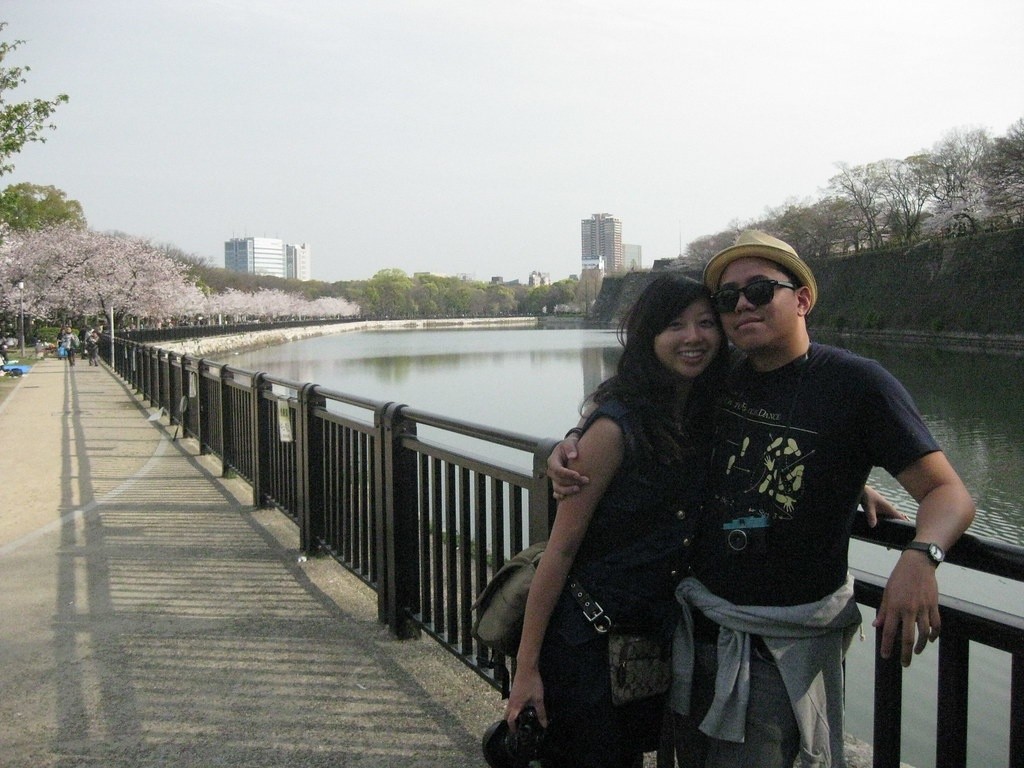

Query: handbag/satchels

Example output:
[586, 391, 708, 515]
[469, 542, 553, 657]
[609, 627, 672, 702]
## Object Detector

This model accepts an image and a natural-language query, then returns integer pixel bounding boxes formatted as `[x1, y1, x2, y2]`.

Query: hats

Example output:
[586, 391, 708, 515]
[704, 228, 818, 317]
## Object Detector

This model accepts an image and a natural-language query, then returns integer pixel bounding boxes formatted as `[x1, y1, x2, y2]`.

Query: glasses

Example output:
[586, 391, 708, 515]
[708, 281, 796, 313]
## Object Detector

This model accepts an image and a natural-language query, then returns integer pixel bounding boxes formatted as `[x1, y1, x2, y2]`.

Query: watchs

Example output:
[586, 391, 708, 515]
[900, 541, 947, 567]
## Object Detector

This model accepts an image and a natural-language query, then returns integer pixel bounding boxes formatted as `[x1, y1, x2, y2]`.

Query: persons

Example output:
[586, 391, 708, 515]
[0, 307, 206, 377]
[542, 232, 979, 768]
[489, 270, 905, 768]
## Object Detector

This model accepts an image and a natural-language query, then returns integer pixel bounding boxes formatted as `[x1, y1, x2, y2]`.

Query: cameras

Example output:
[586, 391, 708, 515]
[483, 706, 548, 768]
